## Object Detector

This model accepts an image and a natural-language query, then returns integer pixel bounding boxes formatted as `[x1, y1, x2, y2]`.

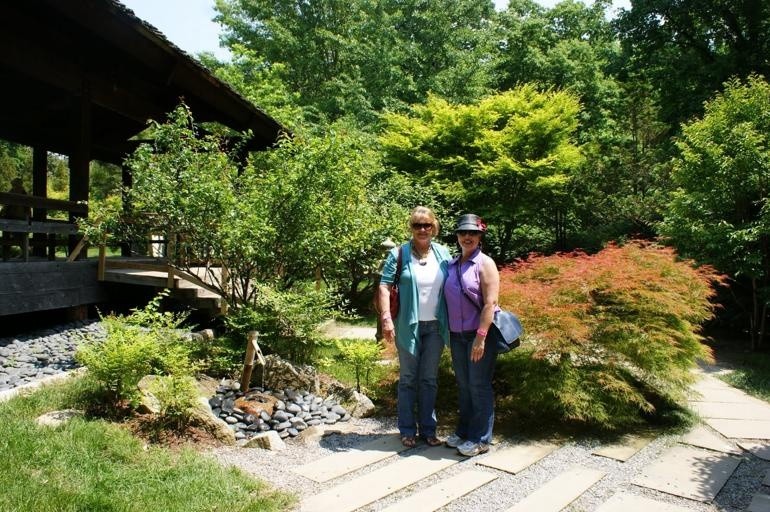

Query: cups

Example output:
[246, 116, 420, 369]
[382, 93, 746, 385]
[413, 223, 432, 231]
[459, 231, 480, 236]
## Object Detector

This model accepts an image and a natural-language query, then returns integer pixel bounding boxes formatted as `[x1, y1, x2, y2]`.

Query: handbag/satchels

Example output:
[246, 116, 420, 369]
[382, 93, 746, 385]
[476, 328, 488, 337]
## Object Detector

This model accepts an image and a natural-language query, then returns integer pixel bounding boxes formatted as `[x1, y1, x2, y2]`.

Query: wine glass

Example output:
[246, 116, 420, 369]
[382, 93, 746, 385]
[478, 352, 482, 354]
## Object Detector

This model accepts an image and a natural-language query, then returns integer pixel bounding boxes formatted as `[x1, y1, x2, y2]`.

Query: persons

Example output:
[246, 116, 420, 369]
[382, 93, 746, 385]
[442, 212, 502, 457]
[377, 205, 455, 449]
[0, 177, 32, 262]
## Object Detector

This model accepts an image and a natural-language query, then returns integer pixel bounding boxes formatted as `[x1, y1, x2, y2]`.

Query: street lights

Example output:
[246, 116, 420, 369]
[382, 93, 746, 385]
[371, 282, 399, 321]
[489, 310, 525, 354]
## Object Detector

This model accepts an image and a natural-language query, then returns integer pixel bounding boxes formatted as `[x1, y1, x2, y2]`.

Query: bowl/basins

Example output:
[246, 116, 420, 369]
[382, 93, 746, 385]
[410, 241, 431, 266]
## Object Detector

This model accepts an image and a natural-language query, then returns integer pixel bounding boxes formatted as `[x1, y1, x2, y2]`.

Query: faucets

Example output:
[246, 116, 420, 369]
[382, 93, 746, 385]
[401, 436, 416, 448]
[426, 435, 441, 446]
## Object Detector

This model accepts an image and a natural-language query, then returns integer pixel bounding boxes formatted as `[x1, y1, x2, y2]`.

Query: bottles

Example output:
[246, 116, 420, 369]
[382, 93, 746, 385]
[453, 213, 486, 232]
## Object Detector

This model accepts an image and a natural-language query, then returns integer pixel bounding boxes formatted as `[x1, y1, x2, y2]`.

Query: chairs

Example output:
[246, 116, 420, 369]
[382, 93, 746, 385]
[445, 432, 490, 457]
